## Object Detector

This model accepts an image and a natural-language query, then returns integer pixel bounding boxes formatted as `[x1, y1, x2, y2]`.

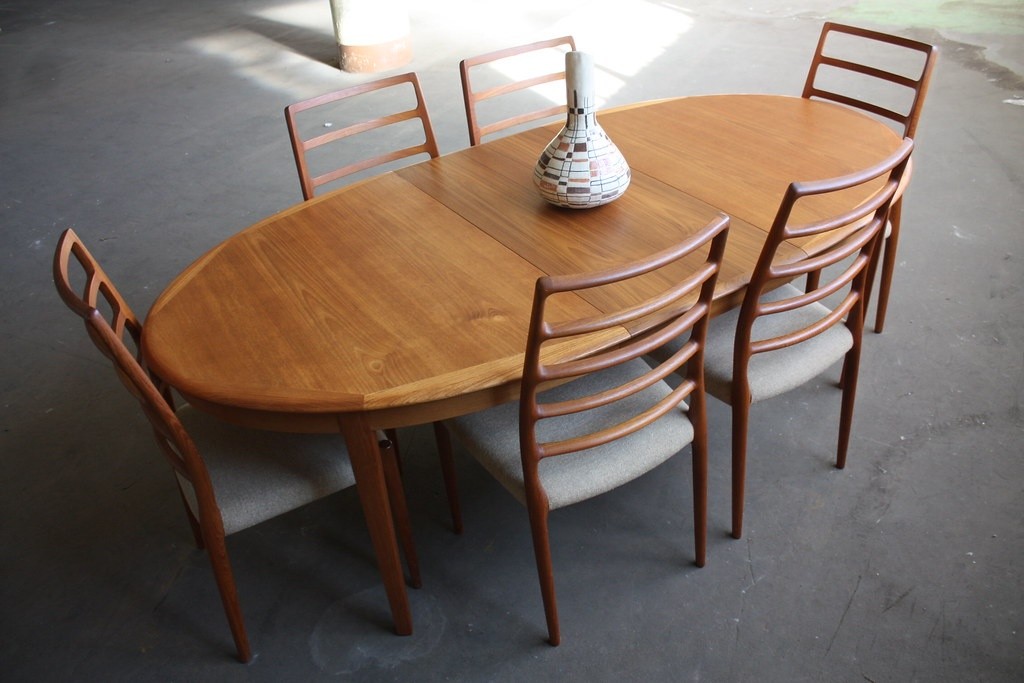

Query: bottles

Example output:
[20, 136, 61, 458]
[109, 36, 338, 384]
[534, 51, 635, 209]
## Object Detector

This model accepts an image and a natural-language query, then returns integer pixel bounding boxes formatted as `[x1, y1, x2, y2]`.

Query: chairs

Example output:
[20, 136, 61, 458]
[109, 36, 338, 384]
[796, 22, 941, 335]
[460, 35, 578, 146]
[631, 137, 913, 540]
[52, 229, 421, 664]
[285, 71, 440, 481]
[427, 213, 709, 648]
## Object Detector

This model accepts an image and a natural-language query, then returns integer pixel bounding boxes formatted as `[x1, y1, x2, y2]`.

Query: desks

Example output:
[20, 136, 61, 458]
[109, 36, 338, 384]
[141, 92, 917, 636]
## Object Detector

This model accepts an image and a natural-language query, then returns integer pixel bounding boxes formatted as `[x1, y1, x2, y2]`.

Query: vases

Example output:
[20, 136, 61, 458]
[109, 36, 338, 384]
[531, 51, 632, 210]
